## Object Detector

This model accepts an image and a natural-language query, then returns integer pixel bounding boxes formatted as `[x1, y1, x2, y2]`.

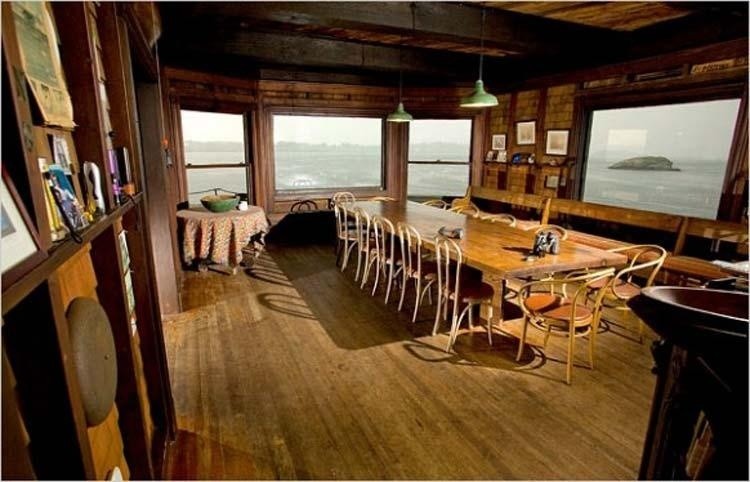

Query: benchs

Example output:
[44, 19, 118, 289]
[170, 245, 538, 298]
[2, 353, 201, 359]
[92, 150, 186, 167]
[450, 185, 750, 298]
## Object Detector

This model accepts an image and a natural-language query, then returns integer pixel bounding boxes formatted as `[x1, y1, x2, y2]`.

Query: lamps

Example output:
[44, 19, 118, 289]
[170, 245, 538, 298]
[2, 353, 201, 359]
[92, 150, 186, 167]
[461, 1, 500, 109]
[386, 36, 413, 123]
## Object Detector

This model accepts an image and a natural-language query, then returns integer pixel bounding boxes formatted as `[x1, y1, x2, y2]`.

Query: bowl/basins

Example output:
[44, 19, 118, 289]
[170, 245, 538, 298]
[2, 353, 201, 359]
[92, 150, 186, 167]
[636, 287, 749, 360]
[200, 193, 241, 213]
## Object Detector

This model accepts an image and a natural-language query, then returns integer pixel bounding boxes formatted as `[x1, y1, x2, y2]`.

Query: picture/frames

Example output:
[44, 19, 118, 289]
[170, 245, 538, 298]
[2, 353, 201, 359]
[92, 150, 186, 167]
[545, 128, 570, 157]
[1, 164, 49, 293]
[491, 132, 506, 151]
[515, 119, 536, 145]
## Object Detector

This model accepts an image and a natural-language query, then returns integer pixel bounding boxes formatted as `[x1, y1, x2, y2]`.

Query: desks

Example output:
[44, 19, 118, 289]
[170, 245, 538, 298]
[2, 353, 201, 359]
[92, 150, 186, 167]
[176, 206, 267, 276]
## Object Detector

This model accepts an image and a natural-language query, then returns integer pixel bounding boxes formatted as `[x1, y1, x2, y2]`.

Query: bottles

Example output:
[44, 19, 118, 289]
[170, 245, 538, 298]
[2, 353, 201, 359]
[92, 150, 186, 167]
[534, 230, 560, 257]
[111, 173, 122, 207]
[438, 227, 465, 239]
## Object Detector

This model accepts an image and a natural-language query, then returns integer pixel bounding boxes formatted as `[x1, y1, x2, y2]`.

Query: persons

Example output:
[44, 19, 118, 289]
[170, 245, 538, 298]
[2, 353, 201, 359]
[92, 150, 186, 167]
[49, 175, 80, 227]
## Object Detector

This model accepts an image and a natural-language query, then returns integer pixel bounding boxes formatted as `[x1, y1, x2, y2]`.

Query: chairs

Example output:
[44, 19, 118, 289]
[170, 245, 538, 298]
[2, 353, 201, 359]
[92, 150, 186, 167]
[329, 192, 628, 351]
[563, 245, 667, 345]
[515, 267, 617, 384]
